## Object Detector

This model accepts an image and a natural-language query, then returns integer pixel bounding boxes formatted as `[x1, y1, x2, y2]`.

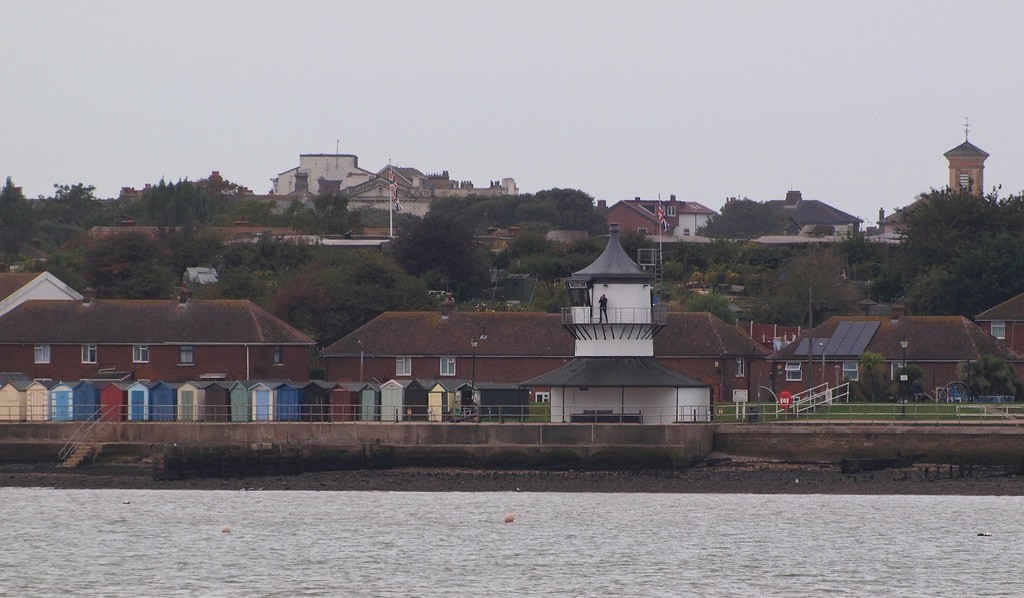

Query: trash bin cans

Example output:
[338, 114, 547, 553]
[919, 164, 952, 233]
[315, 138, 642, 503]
[748, 406, 759, 422]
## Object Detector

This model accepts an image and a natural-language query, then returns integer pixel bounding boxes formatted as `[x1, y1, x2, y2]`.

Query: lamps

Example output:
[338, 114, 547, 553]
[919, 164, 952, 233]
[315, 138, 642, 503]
[715, 362, 721, 373]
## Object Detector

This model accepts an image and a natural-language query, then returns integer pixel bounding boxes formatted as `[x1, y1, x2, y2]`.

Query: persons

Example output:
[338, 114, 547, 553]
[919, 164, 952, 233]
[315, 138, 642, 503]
[598, 295, 608, 323]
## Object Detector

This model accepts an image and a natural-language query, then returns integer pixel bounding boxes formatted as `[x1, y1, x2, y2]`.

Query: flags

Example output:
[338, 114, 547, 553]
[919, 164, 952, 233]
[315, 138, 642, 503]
[658, 205, 670, 232]
[389, 166, 403, 212]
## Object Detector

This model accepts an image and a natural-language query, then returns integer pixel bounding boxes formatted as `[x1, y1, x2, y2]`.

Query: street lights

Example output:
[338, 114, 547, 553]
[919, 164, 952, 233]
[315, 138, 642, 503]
[469, 336, 480, 405]
[898, 334, 910, 414]
